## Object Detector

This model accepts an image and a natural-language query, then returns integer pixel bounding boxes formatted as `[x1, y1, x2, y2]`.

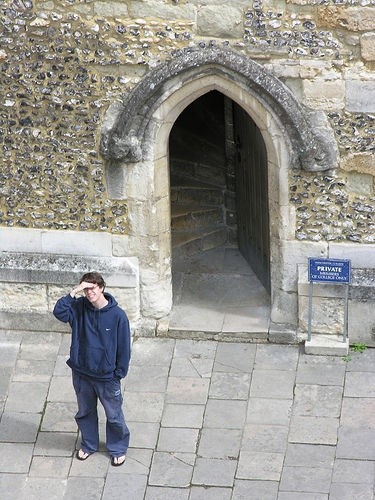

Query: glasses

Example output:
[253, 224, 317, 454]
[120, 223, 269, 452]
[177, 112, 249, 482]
[83, 286, 98, 291]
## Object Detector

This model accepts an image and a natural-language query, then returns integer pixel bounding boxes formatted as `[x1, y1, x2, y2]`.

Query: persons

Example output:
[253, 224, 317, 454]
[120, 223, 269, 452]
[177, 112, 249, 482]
[53, 271, 131, 467]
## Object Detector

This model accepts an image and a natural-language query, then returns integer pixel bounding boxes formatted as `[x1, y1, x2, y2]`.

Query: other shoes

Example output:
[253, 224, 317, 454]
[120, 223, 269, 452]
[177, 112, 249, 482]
[76, 449, 90, 460]
[111, 456, 125, 466]
